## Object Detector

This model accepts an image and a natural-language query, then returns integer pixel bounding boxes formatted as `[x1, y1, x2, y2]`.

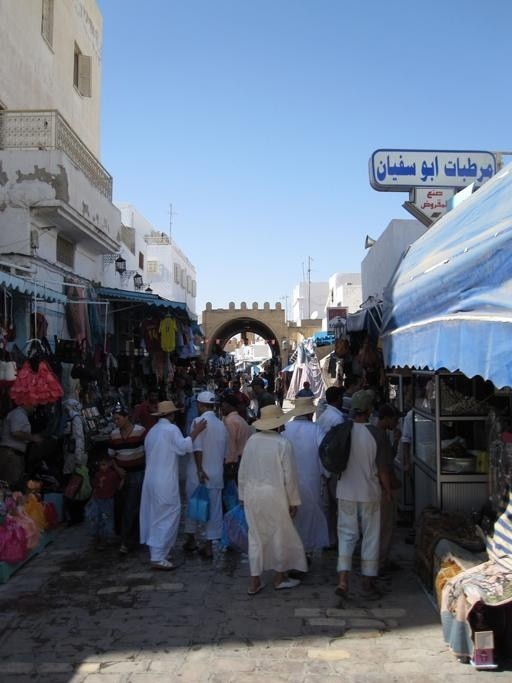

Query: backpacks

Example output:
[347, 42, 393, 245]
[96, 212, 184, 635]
[318, 418, 354, 474]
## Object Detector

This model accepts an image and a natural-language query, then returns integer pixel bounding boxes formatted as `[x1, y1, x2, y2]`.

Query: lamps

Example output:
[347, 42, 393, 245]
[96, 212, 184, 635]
[103, 250, 153, 294]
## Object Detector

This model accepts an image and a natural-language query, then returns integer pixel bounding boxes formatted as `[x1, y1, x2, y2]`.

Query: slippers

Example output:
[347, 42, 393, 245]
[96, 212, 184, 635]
[198, 547, 214, 559]
[246, 579, 268, 595]
[273, 576, 304, 590]
[180, 541, 199, 551]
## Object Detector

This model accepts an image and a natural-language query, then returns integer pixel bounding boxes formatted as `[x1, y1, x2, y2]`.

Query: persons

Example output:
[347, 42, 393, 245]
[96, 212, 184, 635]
[282, 396, 331, 567]
[329, 389, 393, 595]
[131, 354, 386, 429]
[219, 395, 251, 550]
[238, 405, 308, 595]
[107, 408, 147, 553]
[401, 409, 415, 535]
[366, 407, 404, 580]
[89, 454, 125, 552]
[316, 386, 344, 550]
[183, 391, 229, 559]
[0, 402, 45, 486]
[139, 400, 208, 570]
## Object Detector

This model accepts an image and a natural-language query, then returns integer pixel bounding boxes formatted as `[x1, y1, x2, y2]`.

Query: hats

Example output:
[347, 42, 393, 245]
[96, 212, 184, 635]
[325, 385, 347, 401]
[196, 390, 222, 405]
[108, 403, 131, 416]
[286, 396, 320, 416]
[219, 394, 240, 407]
[350, 387, 378, 413]
[247, 377, 265, 388]
[250, 404, 292, 433]
[149, 400, 182, 417]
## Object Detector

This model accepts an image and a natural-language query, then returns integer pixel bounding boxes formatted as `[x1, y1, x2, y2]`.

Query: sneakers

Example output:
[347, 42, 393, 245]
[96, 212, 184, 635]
[87, 535, 178, 570]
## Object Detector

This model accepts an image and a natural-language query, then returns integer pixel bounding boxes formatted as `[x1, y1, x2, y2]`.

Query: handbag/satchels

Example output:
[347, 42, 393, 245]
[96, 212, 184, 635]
[59, 464, 93, 506]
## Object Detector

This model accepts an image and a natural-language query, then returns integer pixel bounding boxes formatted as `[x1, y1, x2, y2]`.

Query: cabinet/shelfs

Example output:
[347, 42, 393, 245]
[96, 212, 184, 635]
[385, 372, 415, 511]
[413, 371, 493, 540]
